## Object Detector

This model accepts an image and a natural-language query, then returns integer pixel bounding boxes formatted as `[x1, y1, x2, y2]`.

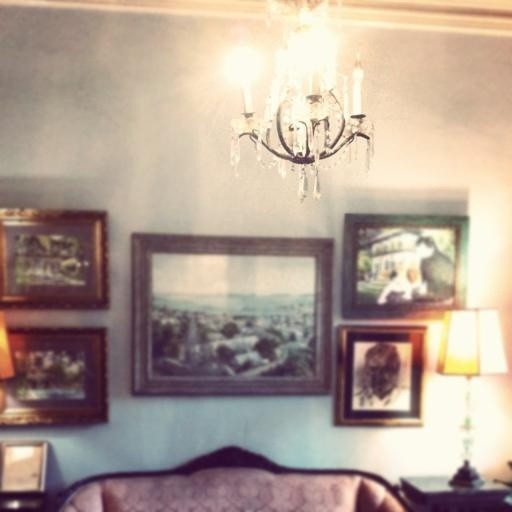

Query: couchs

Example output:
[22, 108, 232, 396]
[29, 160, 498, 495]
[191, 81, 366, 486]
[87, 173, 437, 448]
[51, 446, 416, 512]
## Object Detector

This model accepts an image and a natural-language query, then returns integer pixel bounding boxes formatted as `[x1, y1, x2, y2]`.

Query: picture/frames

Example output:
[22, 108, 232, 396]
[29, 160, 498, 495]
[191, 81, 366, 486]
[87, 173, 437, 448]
[341, 212, 469, 321]
[130, 231, 335, 397]
[1, 437, 50, 493]
[0, 206, 110, 429]
[334, 325, 427, 427]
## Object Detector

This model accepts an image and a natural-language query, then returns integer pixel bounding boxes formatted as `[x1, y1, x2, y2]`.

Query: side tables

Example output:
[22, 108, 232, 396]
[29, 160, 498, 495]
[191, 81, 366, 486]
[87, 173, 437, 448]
[394, 475, 511, 512]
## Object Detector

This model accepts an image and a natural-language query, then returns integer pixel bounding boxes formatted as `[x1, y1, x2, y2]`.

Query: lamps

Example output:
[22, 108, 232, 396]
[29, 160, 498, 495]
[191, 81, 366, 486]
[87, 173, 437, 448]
[438, 309, 509, 489]
[223, 0, 376, 205]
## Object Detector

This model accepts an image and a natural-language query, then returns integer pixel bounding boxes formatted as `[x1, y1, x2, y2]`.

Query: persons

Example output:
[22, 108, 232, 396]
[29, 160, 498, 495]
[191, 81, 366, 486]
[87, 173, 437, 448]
[351, 340, 409, 411]
